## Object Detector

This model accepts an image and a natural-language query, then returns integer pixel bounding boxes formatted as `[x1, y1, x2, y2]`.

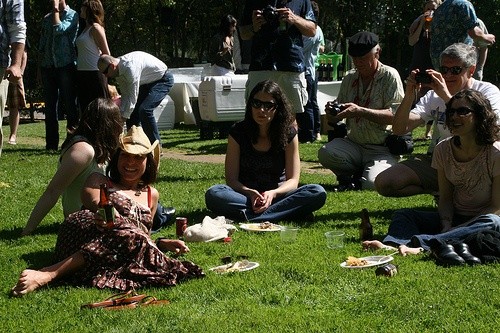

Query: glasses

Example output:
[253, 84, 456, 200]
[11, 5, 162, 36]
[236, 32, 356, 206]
[249, 97, 276, 109]
[438, 65, 470, 74]
[446, 105, 473, 117]
[102, 62, 113, 74]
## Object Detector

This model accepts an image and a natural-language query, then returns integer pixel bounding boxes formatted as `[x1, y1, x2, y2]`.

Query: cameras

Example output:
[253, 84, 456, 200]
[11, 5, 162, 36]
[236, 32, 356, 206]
[259, 5, 281, 23]
[329, 103, 344, 116]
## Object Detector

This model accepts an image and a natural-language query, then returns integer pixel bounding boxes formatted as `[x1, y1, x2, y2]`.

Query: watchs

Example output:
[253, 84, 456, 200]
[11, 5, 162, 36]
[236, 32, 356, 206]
[155, 237, 166, 249]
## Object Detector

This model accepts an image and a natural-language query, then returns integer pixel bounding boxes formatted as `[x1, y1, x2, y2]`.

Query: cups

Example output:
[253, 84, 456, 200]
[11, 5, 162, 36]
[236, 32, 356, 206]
[425, 8, 435, 22]
[280, 226, 299, 243]
[325, 230, 345, 249]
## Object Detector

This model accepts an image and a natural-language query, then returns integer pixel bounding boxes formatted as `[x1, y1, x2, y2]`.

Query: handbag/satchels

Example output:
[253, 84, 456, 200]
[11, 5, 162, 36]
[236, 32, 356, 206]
[386, 133, 415, 155]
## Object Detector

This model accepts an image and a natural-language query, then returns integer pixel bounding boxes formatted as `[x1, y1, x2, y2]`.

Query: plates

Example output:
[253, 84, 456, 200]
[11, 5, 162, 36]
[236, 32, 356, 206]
[211, 261, 259, 274]
[240, 223, 284, 232]
[341, 256, 393, 269]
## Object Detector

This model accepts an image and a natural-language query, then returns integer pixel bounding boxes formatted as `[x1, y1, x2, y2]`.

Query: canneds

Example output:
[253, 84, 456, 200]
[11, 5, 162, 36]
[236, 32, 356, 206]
[175, 217, 187, 236]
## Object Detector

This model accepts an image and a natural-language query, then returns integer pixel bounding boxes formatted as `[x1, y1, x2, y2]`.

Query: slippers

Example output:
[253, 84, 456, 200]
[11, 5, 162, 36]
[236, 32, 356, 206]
[85, 287, 171, 311]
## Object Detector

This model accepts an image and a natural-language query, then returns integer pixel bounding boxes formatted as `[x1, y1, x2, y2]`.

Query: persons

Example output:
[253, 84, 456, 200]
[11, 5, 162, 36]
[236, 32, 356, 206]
[375, 43, 500, 198]
[39, 0, 174, 152]
[203, 81, 326, 227]
[10, 99, 175, 237]
[300, 2, 325, 144]
[7, 52, 28, 145]
[0, 0, 27, 159]
[210, 15, 237, 76]
[361, 88, 500, 257]
[238, 0, 316, 116]
[10, 125, 206, 297]
[317, 32, 413, 187]
[409, 0, 496, 139]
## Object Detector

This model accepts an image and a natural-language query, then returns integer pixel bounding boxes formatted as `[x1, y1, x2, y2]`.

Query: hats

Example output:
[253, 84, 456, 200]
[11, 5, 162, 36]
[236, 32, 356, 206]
[347, 31, 379, 57]
[117, 125, 162, 169]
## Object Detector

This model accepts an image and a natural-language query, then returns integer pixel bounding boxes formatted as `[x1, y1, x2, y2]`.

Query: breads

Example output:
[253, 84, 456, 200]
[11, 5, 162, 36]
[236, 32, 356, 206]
[346, 256, 368, 266]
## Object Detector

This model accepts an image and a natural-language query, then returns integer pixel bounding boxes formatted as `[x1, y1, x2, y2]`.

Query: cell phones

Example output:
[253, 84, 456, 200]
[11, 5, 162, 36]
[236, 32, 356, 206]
[414, 72, 433, 83]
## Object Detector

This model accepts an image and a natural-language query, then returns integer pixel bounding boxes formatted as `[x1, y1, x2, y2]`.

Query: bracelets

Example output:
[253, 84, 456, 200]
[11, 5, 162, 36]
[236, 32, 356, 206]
[53, 8, 59, 13]
[439, 215, 452, 221]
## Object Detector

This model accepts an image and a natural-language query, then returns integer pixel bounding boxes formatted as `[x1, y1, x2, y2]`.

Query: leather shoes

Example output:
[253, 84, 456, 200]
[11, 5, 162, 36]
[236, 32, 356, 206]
[435, 241, 481, 268]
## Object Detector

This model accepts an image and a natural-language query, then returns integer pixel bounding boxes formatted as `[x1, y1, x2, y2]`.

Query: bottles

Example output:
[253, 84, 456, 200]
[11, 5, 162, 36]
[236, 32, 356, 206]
[328, 58, 333, 81]
[318, 60, 324, 81]
[358, 209, 373, 241]
[96, 183, 114, 232]
[322, 59, 328, 81]
[337, 58, 344, 81]
[375, 264, 399, 278]
